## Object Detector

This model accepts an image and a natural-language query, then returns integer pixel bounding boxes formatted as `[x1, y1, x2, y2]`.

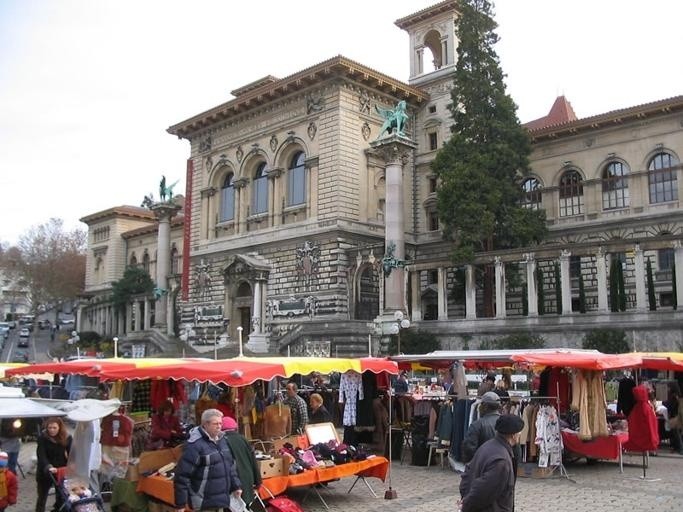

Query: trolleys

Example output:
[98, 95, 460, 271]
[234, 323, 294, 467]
[253, 483, 303, 511]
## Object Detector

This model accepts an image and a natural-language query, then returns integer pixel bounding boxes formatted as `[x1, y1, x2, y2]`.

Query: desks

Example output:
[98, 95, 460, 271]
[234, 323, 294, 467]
[560, 428, 629, 476]
[136, 455, 388, 512]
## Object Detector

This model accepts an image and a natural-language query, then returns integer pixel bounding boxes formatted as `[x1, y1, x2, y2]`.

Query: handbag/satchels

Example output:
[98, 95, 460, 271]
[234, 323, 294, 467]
[186, 477, 204, 511]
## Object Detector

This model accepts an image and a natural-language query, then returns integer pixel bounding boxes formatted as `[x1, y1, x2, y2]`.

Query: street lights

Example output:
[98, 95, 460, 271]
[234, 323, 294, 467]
[66, 328, 79, 357]
[389, 308, 411, 356]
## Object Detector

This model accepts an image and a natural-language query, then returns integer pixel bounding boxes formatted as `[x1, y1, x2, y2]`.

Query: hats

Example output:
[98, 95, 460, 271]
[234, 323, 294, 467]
[221, 417, 238, 431]
[476, 391, 524, 434]
[0, 451, 9, 467]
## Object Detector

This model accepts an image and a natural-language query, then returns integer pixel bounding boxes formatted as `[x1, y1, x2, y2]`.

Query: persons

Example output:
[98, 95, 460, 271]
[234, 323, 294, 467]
[68, 482, 95, 503]
[462, 392, 521, 511]
[143, 401, 188, 453]
[395, 372, 408, 393]
[493, 379, 510, 403]
[0, 436, 22, 479]
[662, 381, 683, 455]
[309, 393, 335, 490]
[217, 416, 261, 511]
[436, 368, 452, 392]
[477, 375, 494, 398]
[35, 418, 77, 511]
[280, 382, 309, 437]
[458, 414, 525, 512]
[0, 451, 17, 511]
[260, 398, 290, 443]
[496, 366, 517, 388]
[172, 409, 244, 511]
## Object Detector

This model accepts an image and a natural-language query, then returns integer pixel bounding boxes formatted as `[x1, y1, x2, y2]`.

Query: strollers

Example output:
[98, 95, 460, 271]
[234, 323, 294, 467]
[48, 465, 107, 511]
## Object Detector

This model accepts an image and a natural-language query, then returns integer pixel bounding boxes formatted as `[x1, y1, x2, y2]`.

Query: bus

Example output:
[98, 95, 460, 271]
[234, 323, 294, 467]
[447, 372, 531, 401]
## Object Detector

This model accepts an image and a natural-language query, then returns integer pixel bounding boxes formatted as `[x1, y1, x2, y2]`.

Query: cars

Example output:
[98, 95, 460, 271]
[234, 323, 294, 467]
[65, 355, 97, 362]
[37, 319, 50, 330]
[0, 314, 35, 364]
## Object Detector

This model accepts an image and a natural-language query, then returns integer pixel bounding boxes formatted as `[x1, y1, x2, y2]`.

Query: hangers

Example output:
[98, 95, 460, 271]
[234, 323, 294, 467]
[201, 387, 214, 401]
[426, 396, 552, 408]
